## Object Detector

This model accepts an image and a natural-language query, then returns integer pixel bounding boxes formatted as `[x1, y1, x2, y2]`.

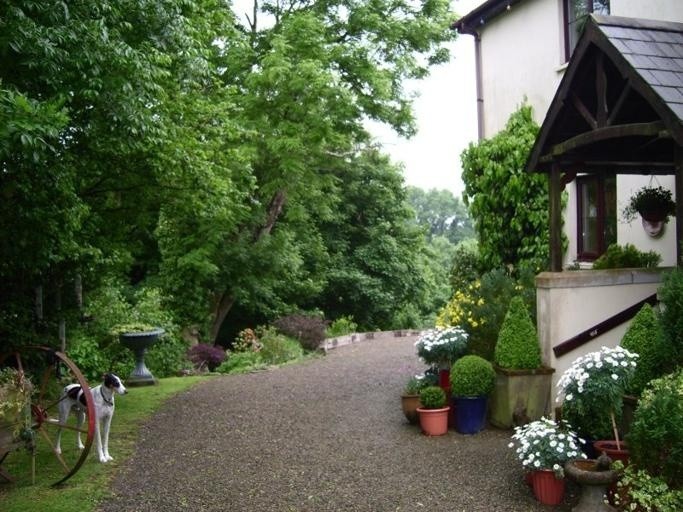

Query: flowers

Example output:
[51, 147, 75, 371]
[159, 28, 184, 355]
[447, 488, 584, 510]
[412, 324, 469, 364]
[503, 346, 640, 470]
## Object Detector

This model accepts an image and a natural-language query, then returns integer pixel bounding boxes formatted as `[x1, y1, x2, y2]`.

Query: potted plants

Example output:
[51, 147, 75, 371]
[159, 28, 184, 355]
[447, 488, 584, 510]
[398, 355, 497, 438]
[488, 296, 557, 429]
[115, 322, 166, 385]
[617, 175, 679, 226]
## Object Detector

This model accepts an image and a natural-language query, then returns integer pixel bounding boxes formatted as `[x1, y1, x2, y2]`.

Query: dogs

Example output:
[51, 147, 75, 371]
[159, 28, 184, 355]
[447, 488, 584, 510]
[55, 373, 128, 463]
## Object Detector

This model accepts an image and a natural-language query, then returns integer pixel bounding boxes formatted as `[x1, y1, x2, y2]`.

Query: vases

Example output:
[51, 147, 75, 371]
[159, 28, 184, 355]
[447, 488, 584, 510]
[592, 436, 633, 467]
[529, 467, 564, 505]
